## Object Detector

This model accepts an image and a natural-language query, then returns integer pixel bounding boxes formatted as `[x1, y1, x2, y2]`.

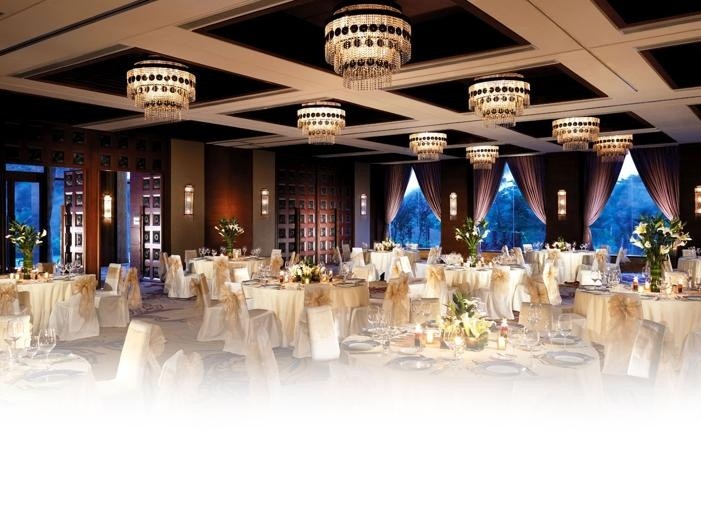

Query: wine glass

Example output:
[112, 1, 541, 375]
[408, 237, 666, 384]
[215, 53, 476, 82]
[590, 265, 650, 292]
[439, 255, 504, 268]
[364, 296, 573, 367]
[197, 246, 262, 260]
[256, 259, 355, 286]
[56, 259, 82, 282]
[580, 242, 591, 252]
[3, 318, 57, 367]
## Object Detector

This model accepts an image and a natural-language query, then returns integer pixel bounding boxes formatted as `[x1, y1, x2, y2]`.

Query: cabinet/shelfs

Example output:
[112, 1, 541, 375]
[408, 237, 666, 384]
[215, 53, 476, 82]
[140, 172, 164, 284]
[64, 169, 117, 274]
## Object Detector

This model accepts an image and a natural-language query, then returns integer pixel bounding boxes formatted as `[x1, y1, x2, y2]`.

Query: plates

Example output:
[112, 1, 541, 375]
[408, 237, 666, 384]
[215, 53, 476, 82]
[342, 319, 594, 375]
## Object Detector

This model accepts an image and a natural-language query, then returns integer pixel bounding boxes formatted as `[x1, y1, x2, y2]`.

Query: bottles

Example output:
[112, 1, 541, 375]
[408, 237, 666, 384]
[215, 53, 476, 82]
[672, 283, 683, 294]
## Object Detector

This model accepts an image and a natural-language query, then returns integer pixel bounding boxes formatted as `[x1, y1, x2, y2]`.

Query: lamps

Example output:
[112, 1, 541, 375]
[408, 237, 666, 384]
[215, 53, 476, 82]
[323, 0, 412, 91]
[104, 195, 112, 218]
[409, 118, 634, 171]
[467, 72, 531, 128]
[297, 99, 346, 145]
[125, 55, 197, 121]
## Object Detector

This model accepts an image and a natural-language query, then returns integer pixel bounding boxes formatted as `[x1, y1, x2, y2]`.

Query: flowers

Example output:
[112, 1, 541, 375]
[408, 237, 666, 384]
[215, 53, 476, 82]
[5, 220, 47, 253]
[628, 213, 693, 261]
[213, 216, 244, 242]
[551, 236, 571, 250]
[381, 236, 395, 246]
[454, 215, 491, 248]
